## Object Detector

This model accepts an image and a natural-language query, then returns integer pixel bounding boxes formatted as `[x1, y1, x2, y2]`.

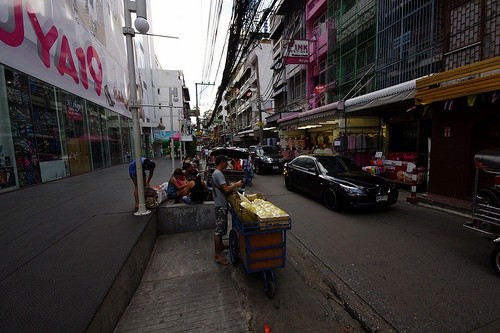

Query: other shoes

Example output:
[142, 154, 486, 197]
[216, 258, 230, 265]
[220, 245, 230, 251]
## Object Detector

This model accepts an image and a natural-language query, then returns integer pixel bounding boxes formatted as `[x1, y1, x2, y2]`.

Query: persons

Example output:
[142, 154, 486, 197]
[182, 146, 213, 169]
[280, 147, 312, 161]
[129, 158, 156, 207]
[167, 168, 191, 203]
[182, 169, 208, 204]
[211, 154, 243, 265]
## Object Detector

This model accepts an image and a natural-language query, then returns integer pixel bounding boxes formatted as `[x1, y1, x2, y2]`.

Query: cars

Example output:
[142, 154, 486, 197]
[283, 154, 398, 210]
[252, 145, 286, 174]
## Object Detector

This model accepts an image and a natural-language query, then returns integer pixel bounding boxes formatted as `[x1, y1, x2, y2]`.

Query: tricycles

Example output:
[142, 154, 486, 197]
[229, 205, 292, 301]
